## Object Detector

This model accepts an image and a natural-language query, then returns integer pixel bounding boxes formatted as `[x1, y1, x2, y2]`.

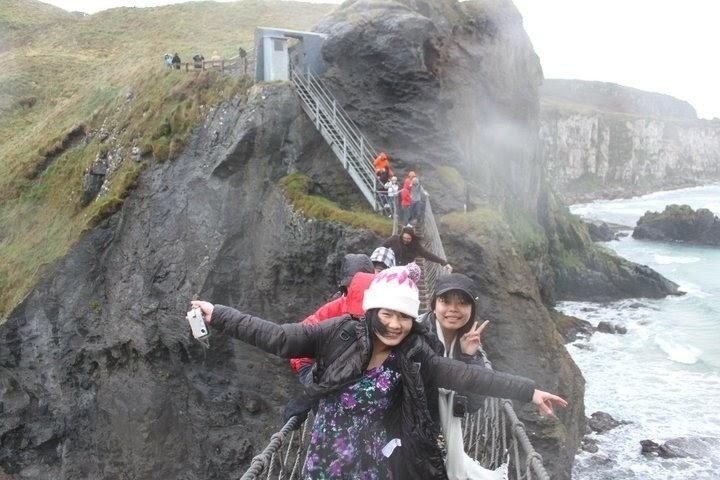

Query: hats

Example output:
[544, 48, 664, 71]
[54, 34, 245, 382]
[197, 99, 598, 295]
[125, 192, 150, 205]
[362, 265, 421, 321]
[435, 272, 478, 305]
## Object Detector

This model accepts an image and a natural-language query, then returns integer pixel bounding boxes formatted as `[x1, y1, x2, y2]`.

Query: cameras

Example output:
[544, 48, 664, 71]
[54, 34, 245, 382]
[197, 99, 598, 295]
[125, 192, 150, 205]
[453, 394, 467, 418]
[185, 307, 212, 340]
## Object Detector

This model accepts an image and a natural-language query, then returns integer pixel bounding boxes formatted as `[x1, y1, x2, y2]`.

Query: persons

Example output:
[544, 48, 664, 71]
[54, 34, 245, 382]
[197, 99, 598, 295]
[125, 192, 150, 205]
[329, 251, 377, 301]
[415, 273, 493, 479]
[239, 47, 250, 66]
[373, 152, 430, 233]
[288, 271, 378, 388]
[163, 52, 173, 68]
[211, 51, 222, 71]
[189, 262, 569, 480]
[193, 54, 205, 69]
[368, 245, 397, 275]
[172, 53, 181, 69]
[380, 226, 453, 272]
